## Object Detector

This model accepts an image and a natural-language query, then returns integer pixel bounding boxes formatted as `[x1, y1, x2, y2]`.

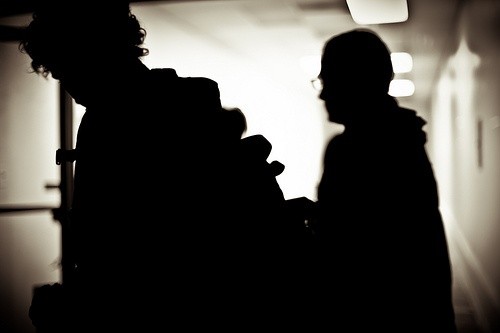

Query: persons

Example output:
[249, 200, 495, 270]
[15, 0, 291, 333]
[294, 27, 460, 333]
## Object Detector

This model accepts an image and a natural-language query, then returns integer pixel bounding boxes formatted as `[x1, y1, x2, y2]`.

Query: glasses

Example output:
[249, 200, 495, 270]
[310, 76, 323, 90]
